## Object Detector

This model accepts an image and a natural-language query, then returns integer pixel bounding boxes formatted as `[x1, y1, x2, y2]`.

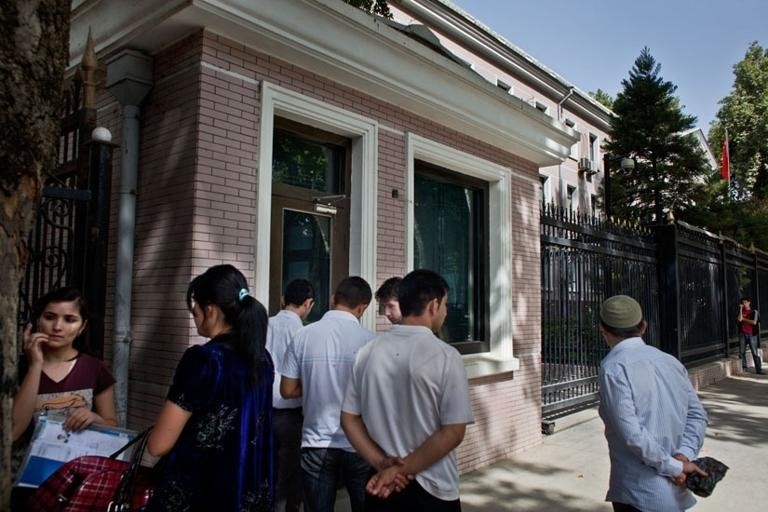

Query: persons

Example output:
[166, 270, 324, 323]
[266, 279, 317, 507]
[339, 271, 485, 511]
[280, 277, 368, 511]
[375, 278, 406, 326]
[735, 297, 765, 374]
[143, 264, 275, 511]
[13, 288, 120, 509]
[594, 296, 708, 511]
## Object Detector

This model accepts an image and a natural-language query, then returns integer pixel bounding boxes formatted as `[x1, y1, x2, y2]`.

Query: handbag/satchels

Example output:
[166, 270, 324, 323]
[106, 423, 193, 512]
[30, 422, 159, 511]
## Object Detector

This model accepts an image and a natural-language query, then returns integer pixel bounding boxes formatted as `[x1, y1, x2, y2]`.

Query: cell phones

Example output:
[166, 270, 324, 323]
[23, 318, 36, 336]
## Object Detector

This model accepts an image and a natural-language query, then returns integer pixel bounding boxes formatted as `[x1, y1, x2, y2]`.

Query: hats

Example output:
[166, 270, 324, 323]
[598, 296, 644, 330]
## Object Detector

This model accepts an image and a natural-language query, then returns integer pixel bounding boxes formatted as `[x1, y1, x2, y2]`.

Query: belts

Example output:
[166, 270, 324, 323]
[272, 407, 303, 414]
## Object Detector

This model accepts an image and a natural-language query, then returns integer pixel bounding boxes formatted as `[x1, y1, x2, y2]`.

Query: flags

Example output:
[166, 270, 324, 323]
[722, 134, 728, 179]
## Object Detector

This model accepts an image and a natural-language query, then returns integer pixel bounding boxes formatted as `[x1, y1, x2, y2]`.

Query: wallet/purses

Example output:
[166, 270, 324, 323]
[688, 457, 729, 498]
